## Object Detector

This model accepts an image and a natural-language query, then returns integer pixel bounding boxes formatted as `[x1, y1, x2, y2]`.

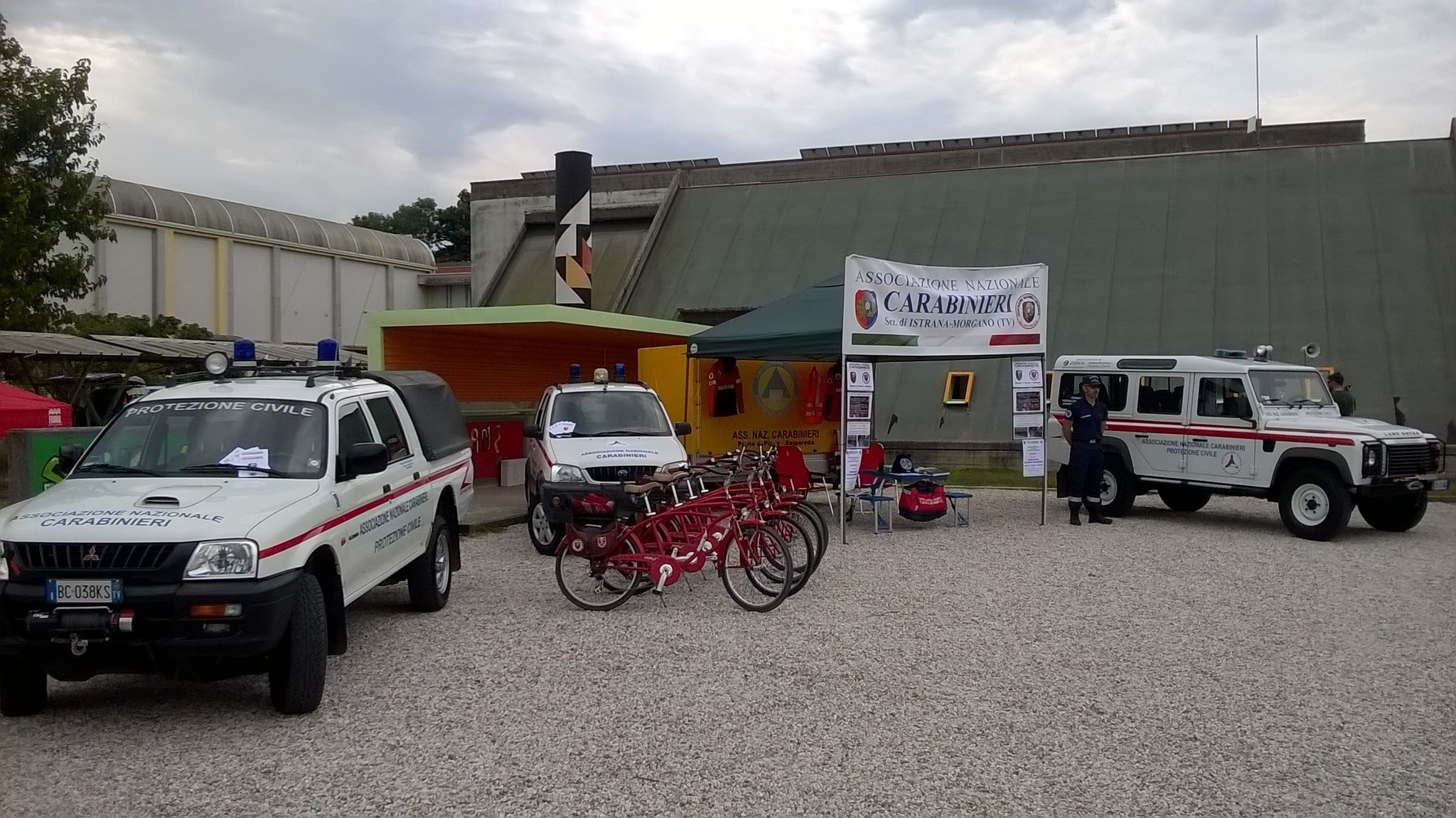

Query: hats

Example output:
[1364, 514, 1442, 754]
[1083, 375, 1101, 385]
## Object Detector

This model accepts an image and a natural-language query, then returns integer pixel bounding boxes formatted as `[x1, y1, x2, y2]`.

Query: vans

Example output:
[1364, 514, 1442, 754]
[524, 363, 694, 554]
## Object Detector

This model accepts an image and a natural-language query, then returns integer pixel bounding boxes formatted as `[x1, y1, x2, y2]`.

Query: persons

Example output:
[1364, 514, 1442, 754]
[1326, 372, 1356, 417]
[1062, 375, 1113, 526]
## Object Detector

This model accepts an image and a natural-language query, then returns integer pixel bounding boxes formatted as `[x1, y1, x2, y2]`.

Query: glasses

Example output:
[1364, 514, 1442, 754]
[1327, 380, 1333, 384]
[1084, 384, 1101, 389]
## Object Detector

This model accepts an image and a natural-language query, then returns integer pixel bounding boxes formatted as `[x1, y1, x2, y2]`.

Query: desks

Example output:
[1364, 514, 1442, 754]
[863, 466, 951, 514]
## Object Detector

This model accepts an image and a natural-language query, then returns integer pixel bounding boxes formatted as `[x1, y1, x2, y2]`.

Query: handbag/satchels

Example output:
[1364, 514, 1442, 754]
[898, 480, 948, 522]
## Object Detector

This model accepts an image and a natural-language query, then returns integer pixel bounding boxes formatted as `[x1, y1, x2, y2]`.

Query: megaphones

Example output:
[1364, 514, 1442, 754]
[1301, 342, 1321, 359]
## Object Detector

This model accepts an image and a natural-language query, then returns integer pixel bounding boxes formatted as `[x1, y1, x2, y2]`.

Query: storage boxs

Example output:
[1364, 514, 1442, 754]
[501, 458, 527, 486]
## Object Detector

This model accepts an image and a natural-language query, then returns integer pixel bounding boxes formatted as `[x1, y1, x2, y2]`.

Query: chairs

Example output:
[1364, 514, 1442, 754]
[773, 446, 834, 517]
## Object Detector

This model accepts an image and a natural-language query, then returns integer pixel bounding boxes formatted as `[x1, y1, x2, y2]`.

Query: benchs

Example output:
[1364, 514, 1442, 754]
[945, 490, 973, 528]
[858, 495, 893, 534]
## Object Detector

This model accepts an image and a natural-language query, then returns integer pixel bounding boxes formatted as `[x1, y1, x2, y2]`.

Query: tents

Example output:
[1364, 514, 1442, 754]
[684, 273, 1045, 546]
[0, 382, 73, 439]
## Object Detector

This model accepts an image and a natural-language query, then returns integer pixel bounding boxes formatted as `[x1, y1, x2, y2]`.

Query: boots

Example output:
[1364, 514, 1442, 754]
[1087, 496, 1112, 524]
[1067, 496, 1082, 526]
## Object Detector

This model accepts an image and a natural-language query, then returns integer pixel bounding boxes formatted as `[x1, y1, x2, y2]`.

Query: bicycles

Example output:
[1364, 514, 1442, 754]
[555, 445, 828, 612]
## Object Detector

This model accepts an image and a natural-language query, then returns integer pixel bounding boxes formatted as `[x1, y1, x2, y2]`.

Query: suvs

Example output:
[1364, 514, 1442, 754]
[1047, 346, 1447, 541]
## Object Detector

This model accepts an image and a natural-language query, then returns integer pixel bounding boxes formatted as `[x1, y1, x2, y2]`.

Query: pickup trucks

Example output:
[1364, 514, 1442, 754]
[0, 338, 477, 719]
